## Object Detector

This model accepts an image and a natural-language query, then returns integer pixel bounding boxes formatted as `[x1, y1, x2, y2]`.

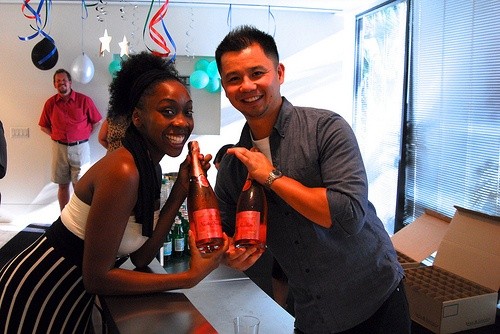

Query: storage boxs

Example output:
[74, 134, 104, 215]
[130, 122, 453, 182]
[389, 204, 500, 334]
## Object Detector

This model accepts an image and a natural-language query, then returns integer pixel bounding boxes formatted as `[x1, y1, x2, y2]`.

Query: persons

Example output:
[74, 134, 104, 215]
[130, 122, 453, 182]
[97, 102, 163, 241]
[0, 52, 229, 334]
[39, 68, 102, 212]
[213, 25, 412, 334]
[213, 144, 289, 308]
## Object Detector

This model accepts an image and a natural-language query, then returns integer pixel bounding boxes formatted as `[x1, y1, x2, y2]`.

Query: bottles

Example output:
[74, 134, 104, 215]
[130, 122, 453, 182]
[159, 179, 169, 208]
[173, 216, 185, 262]
[186, 142, 227, 253]
[235, 148, 268, 248]
[163, 230, 172, 264]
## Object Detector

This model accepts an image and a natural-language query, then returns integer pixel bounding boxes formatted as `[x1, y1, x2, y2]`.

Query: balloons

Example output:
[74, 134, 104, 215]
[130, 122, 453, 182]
[72, 52, 95, 85]
[31, 38, 59, 71]
[190, 58, 223, 94]
[106, 57, 131, 78]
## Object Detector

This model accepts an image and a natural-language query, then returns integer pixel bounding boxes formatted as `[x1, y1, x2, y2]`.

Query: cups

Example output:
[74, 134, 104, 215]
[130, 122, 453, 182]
[233, 316, 259, 334]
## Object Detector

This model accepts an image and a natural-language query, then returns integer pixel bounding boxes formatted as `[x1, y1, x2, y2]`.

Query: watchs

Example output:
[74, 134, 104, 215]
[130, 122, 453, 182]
[265, 170, 283, 186]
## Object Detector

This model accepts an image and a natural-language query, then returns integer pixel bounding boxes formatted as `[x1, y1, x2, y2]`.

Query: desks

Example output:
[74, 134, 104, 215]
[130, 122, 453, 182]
[91, 250, 297, 333]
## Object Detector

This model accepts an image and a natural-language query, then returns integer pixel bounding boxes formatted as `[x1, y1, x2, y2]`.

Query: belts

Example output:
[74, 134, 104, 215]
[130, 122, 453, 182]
[53, 138, 88, 146]
